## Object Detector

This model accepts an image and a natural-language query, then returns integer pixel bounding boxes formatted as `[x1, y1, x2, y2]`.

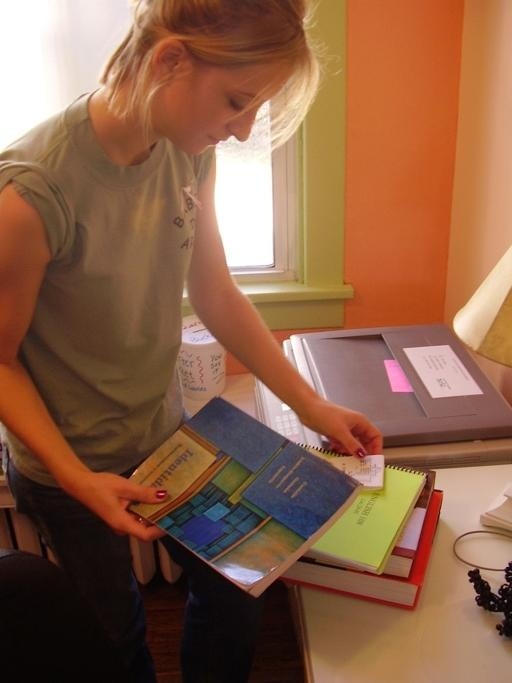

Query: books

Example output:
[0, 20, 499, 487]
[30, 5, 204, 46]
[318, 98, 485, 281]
[126, 396, 364, 599]
[278, 442, 445, 609]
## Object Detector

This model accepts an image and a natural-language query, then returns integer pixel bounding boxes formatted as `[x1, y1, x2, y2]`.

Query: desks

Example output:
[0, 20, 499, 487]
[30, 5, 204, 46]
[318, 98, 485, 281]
[179, 330, 512, 466]
[292, 457, 512, 683]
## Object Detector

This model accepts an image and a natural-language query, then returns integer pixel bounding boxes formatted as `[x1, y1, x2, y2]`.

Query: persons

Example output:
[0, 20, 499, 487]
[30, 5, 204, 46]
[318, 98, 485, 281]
[1, 0, 383, 683]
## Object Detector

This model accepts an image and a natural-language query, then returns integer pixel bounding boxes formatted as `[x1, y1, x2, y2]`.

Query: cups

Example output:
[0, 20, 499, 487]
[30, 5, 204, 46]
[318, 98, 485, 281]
[175, 314, 228, 402]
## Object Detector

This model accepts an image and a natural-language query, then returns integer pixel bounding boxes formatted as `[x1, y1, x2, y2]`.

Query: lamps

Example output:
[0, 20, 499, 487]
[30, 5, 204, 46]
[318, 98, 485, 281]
[452, 243, 511, 533]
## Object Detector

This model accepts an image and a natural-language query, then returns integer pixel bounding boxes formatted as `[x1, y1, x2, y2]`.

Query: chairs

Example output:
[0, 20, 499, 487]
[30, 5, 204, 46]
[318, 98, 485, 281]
[0, 549, 130, 682]
